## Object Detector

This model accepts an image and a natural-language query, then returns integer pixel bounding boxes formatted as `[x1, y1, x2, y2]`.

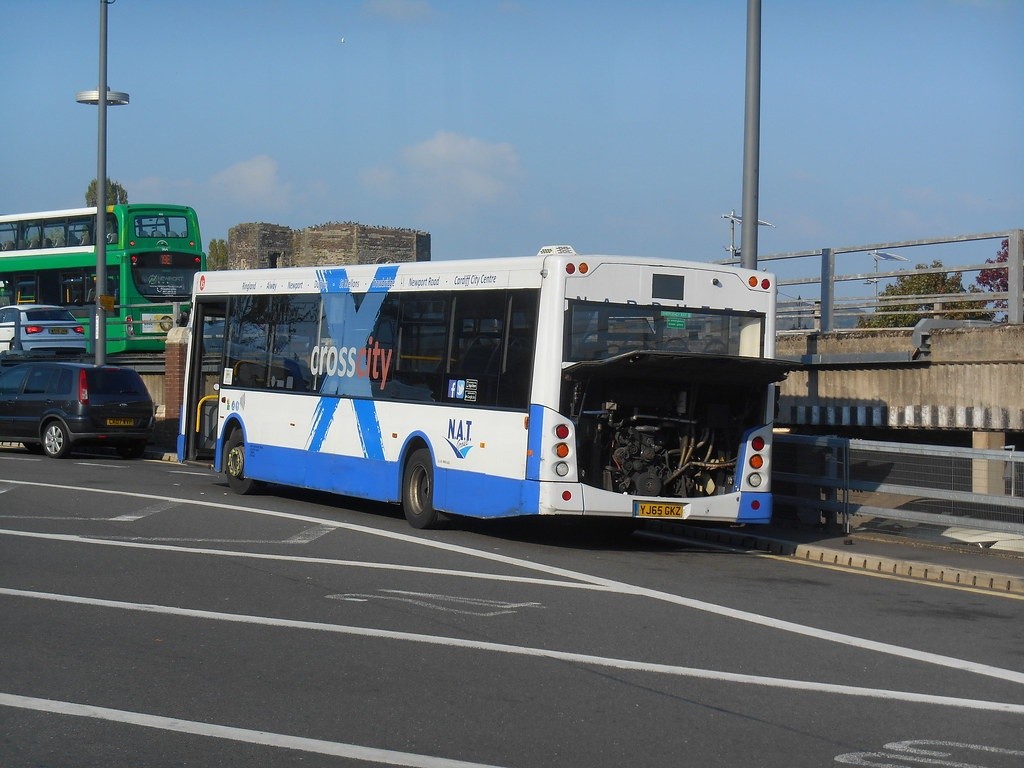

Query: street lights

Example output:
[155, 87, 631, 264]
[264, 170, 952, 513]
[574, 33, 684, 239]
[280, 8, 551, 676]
[720, 210, 775, 268]
[867, 250, 909, 329]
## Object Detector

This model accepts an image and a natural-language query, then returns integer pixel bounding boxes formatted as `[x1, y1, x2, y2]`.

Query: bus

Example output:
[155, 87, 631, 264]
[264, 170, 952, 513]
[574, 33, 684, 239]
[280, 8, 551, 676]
[0, 201, 207, 357]
[173, 244, 808, 529]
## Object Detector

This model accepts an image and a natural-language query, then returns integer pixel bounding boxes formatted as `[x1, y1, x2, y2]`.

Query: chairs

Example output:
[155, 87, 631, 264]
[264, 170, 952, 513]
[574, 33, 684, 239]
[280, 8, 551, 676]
[456, 338, 727, 373]
[0, 229, 187, 252]
[86, 287, 97, 302]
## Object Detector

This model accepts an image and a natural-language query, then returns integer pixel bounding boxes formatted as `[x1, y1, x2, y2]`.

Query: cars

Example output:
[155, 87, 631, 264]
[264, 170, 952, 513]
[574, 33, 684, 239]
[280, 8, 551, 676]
[0, 304, 88, 356]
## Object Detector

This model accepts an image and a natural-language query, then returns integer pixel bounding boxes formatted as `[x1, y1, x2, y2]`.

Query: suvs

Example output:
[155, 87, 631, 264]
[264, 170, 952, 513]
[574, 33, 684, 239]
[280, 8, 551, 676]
[0, 361, 155, 458]
